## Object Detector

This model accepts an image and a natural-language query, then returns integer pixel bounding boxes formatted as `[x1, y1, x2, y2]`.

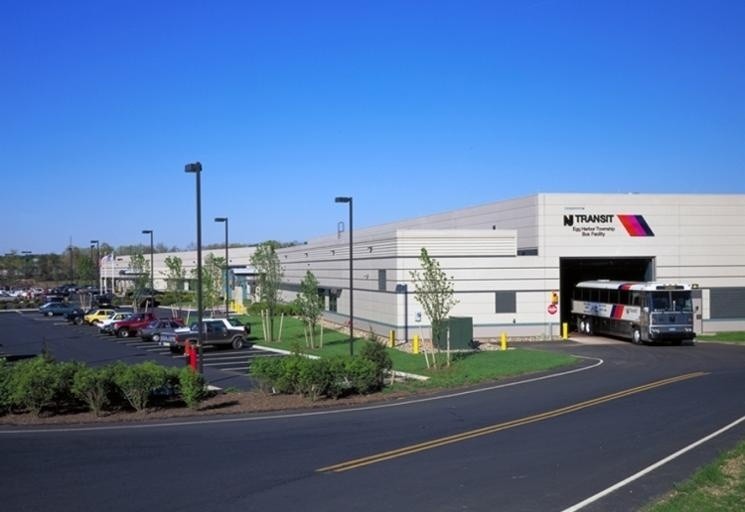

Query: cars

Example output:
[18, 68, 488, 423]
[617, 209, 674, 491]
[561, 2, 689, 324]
[137, 316, 250, 352]
[0, 284, 156, 337]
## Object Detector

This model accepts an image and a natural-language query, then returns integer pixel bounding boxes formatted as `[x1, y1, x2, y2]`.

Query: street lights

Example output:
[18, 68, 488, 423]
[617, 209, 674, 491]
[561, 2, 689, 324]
[67, 240, 101, 292]
[184, 163, 203, 372]
[336, 197, 354, 356]
[215, 218, 229, 317]
[142, 230, 154, 307]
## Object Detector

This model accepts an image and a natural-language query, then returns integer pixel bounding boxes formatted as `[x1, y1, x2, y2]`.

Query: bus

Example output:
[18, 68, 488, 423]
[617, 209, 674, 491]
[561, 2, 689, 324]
[570, 278, 695, 345]
